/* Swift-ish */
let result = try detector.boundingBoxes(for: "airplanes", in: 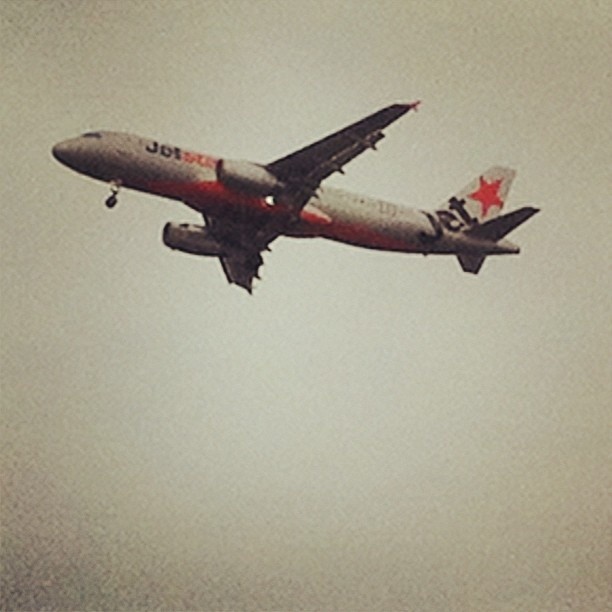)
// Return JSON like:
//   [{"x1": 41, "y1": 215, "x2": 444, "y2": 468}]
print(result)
[{"x1": 53, "y1": 104, "x2": 542, "y2": 294}]
